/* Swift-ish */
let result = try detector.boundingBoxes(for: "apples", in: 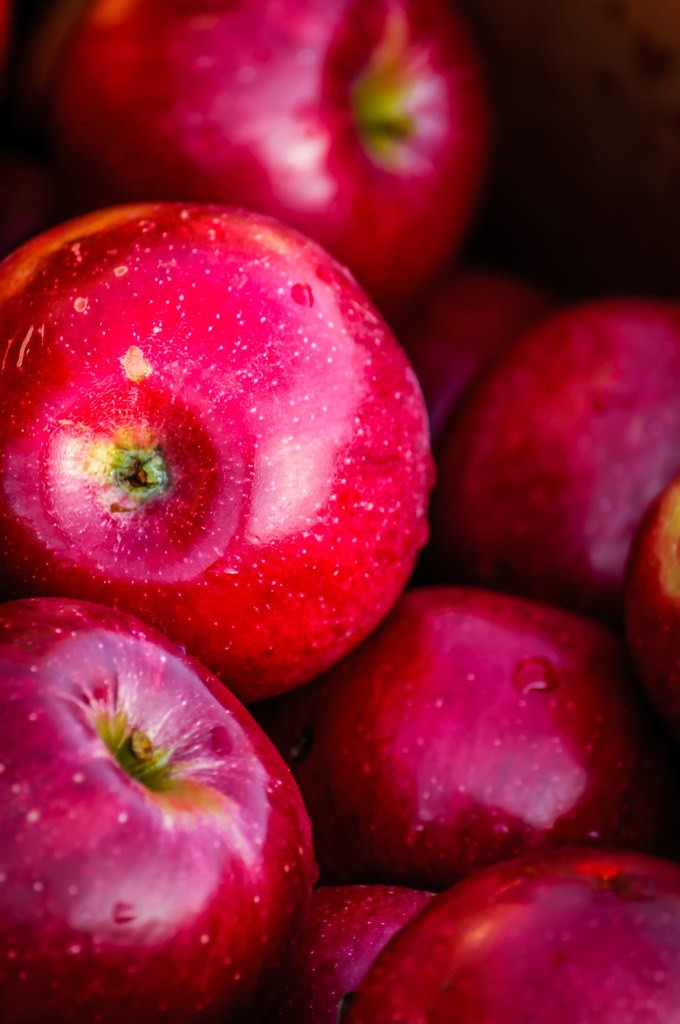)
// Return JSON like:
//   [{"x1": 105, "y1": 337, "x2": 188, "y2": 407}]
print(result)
[{"x1": 0, "y1": 0, "x2": 680, "y2": 1024}]
